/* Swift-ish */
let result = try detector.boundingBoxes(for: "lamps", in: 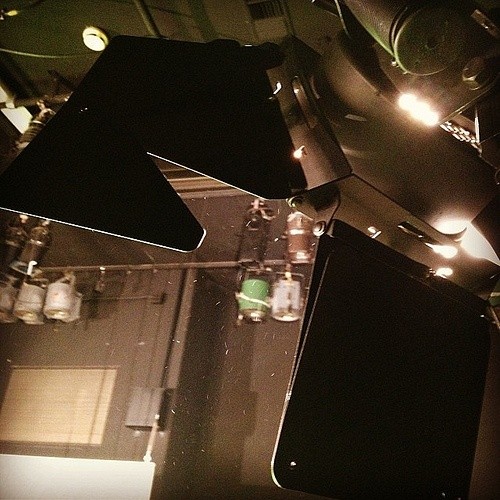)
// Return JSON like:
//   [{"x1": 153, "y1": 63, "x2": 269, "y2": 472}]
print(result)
[
  {"x1": 12, "y1": 279, "x2": 49, "y2": 326},
  {"x1": 44, "y1": 276, "x2": 83, "y2": 322},
  {"x1": 270, "y1": 272, "x2": 306, "y2": 322},
  {"x1": 235, "y1": 271, "x2": 272, "y2": 322},
  {"x1": 287, "y1": 212, "x2": 312, "y2": 259},
  {"x1": 239, "y1": 215, "x2": 271, "y2": 267},
  {"x1": 20, "y1": 121, "x2": 44, "y2": 146},
  {"x1": 19, "y1": 226, "x2": 51, "y2": 264},
  {"x1": 1, "y1": 276, "x2": 20, "y2": 322},
  {"x1": 0, "y1": 219, "x2": 29, "y2": 264}
]
